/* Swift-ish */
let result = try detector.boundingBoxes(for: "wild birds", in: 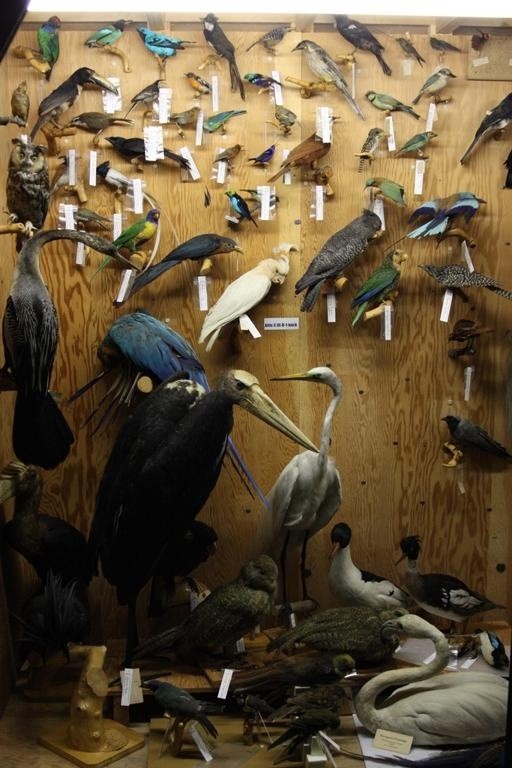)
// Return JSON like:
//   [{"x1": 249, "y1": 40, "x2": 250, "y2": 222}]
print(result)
[{"x1": 0, "y1": 0, "x2": 512, "y2": 766}]
